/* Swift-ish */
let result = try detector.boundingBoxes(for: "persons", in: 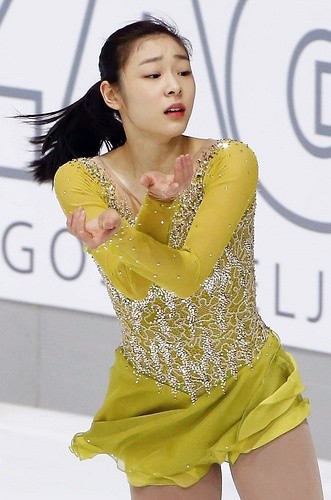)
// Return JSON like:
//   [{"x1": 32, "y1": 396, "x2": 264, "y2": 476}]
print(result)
[{"x1": 8, "y1": 21, "x2": 324, "y2": 500}]
